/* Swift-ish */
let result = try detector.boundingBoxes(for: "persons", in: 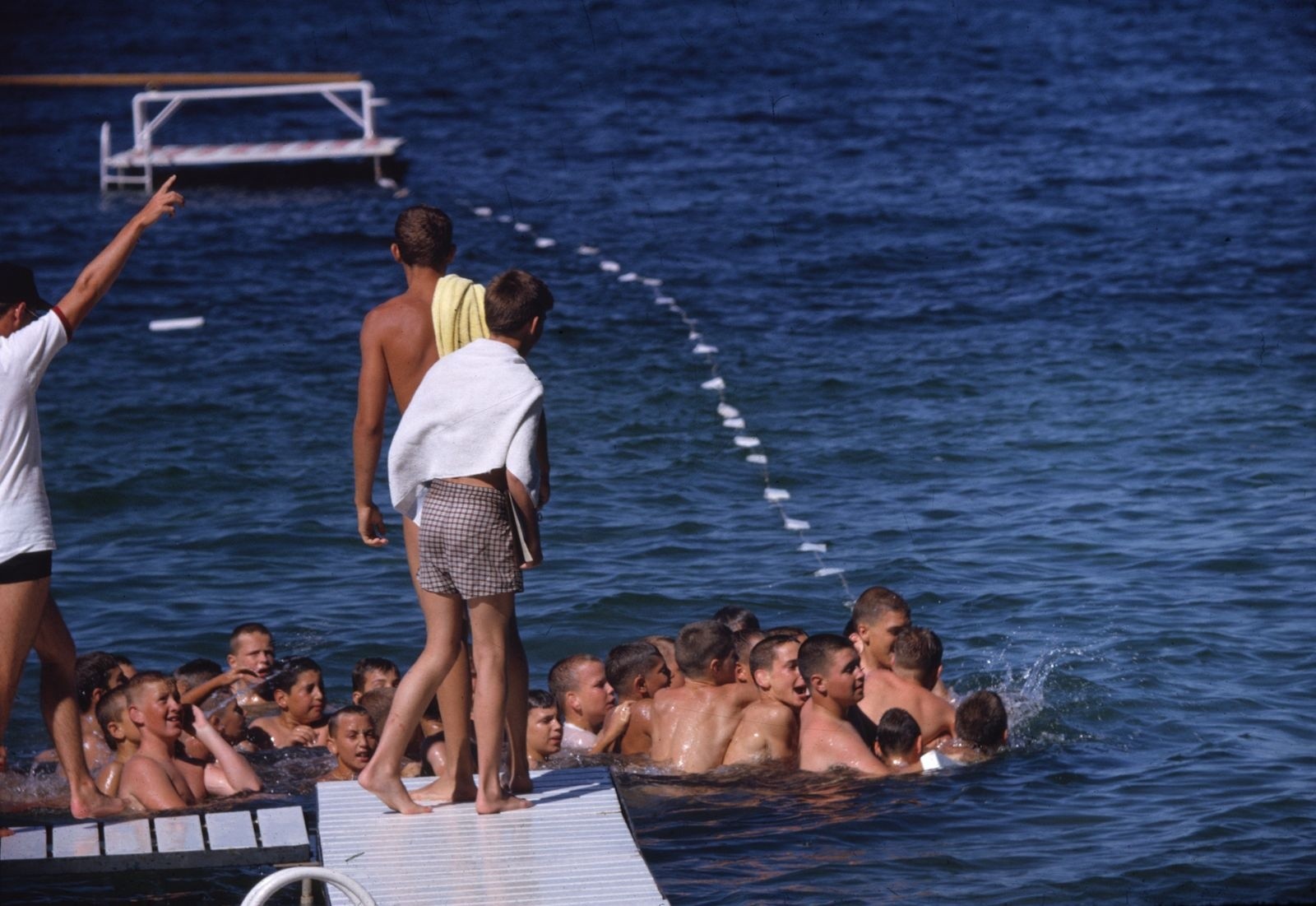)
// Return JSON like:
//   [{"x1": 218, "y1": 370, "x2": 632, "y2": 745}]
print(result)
[
  {"x1": 1, "y1": 175, "x2": 183, "y2": 837},
  {"x1": 352, "y1": 205, "x2": 550, "y2": 804},
  {"x1": 358, "y1": 269, "x2": 554, "y2": 815},
  {"x1": 0, "y1": 586, "x2": 1007, "y2": 818}
]
